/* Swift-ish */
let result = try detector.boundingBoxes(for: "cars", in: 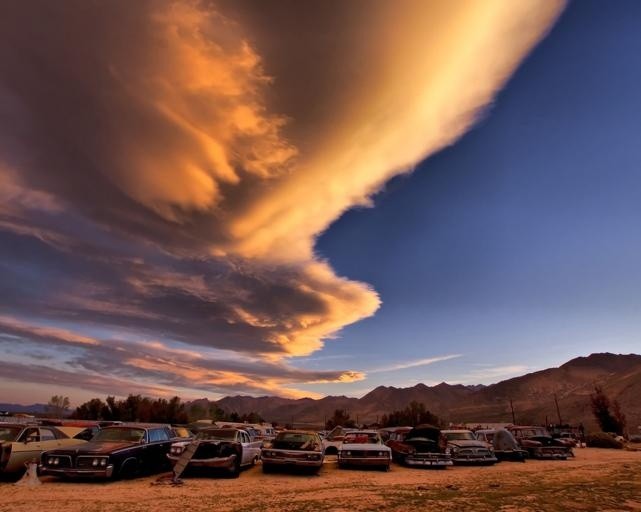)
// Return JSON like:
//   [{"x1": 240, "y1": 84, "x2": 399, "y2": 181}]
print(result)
[
  {"x1": 0, "y1": 420, "x2": 87, "y2": 482},
  {"x1": 605, "y1": 433, "x2": 623, "y2": 443},
  {"x1": 36, "y1": 418, "x2": 124, "y2": 442},
  {"x1": 12, "y1": 413, "x2": 35, "y2": 418},
  {"x1": 32, "y1": 419, "x2": 176, "y2": 484}
]
